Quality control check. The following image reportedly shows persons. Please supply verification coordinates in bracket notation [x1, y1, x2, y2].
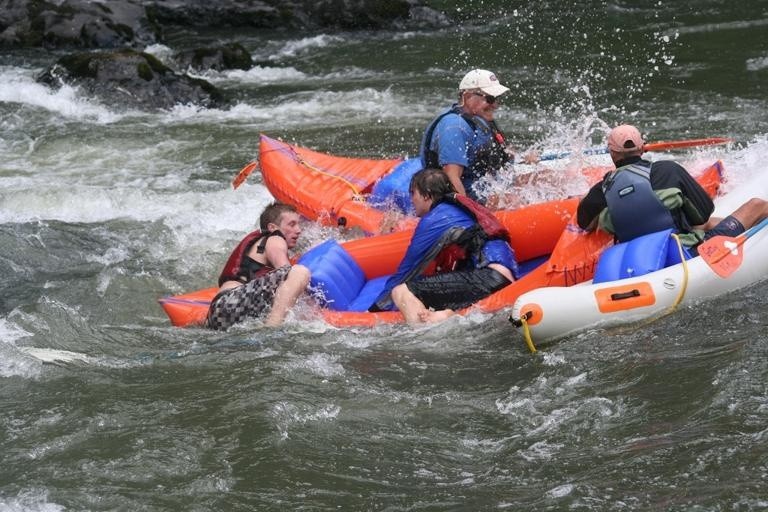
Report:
[419, 68, 542, 206]
[203, 200, 311, 330]
[364, 167, 520, 325]
[575, 123, 768, 256]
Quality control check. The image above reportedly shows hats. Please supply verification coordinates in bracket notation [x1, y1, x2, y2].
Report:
[459, 69, 509, 97]
[608, 124, 644, 151]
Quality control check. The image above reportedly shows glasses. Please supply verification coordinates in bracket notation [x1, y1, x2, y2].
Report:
[473, 92, 495, 104]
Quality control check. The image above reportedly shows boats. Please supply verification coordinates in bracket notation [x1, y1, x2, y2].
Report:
[508, 216, 767, 342]
[155, 192, 615, 332]
[259, 133, 724, 238]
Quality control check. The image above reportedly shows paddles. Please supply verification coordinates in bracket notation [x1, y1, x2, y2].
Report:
[541, 136, 733, 159]
[698, 217, 768, 277]
